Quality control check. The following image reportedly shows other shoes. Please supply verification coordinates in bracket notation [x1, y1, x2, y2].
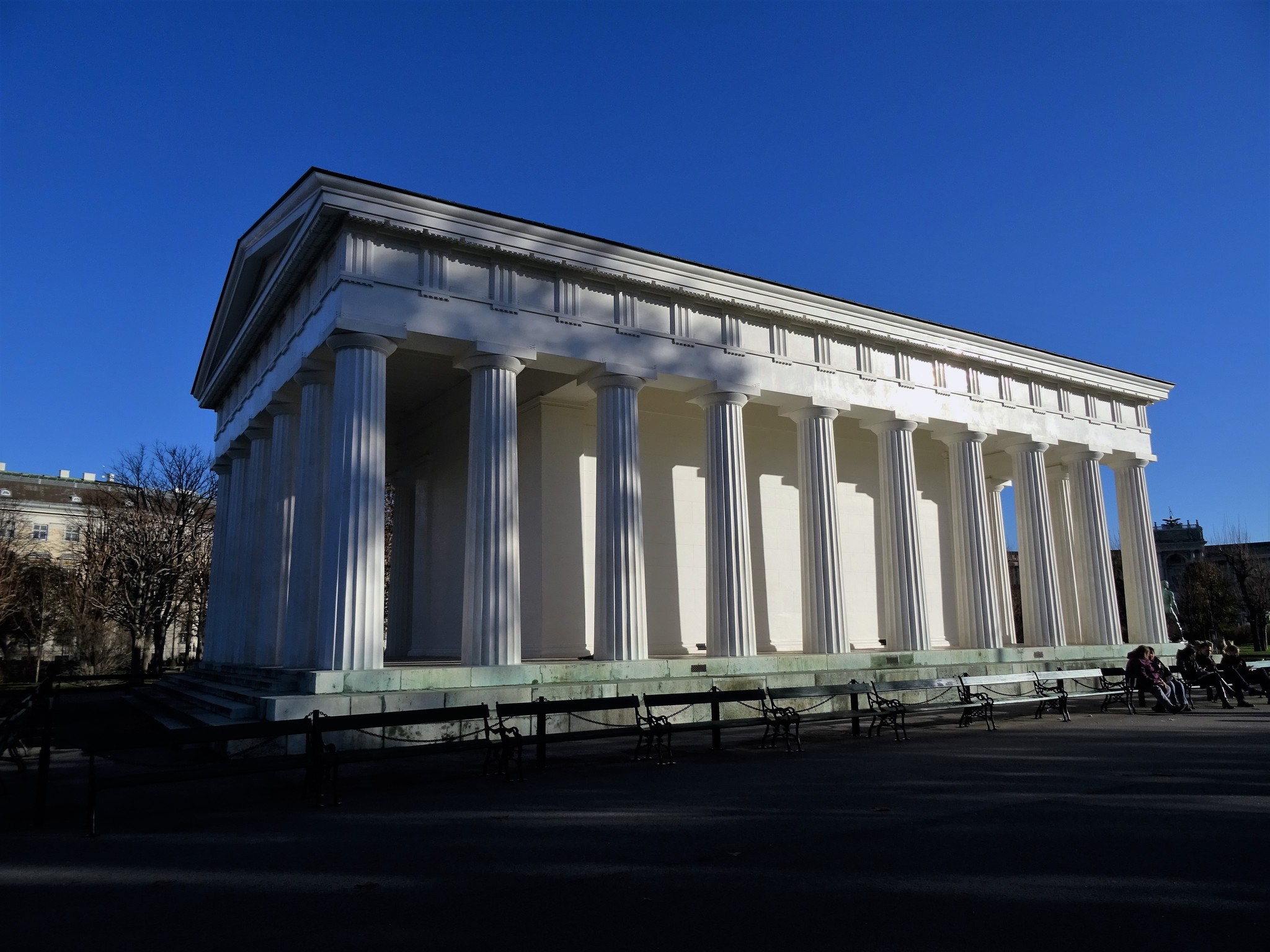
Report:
[1268, 698, 1270, 704]
[1237, 700, 1255, 707]
[1171, 705, 1186, 715]
[1248, 687, 1266, 696]
[1152, 705, 1166, 712]
[1184, 706, 1192, 712]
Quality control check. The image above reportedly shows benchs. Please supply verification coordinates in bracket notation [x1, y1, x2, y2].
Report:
[307, 657, 1269, 798]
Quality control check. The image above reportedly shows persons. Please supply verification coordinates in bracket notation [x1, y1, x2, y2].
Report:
[1177, 640, 1270, 709]
[1124, 645, 1192, 714]
[1161, 580, 1189, 643]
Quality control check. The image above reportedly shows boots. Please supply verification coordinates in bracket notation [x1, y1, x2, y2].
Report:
[1220, 678, 1237, 699]
[1217, 690, 1235, 709]
